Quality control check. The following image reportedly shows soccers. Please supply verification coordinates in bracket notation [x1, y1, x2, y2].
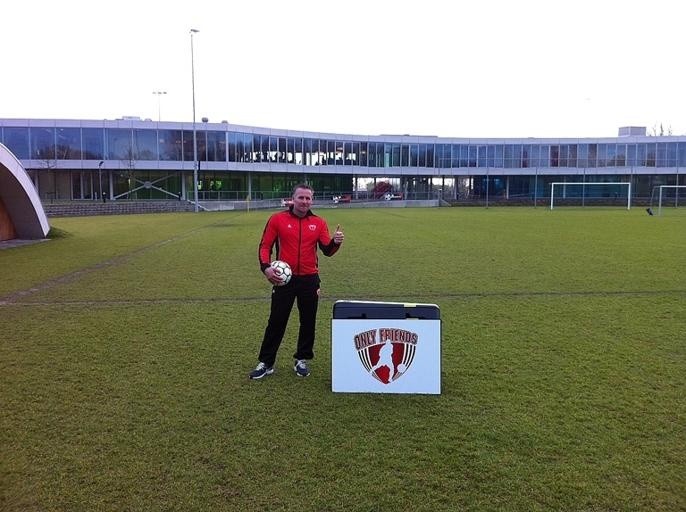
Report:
[270, 260, 292, 286]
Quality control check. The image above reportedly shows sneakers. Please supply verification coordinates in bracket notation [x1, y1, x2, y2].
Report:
[248, 362, 274, 379]
[294, 359, 310, 377]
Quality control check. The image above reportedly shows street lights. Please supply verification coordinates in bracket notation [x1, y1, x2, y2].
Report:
[98, 159, 106, 204]
[189, 28, 201, 213]
[151, 91, 168, 121]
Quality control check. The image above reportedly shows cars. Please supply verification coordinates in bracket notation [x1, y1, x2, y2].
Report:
[280, 198, 293, 207]
[384, 192, 405, 201]
[332, 194, 352, 204]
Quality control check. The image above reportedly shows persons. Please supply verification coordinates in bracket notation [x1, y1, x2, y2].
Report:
[247, 185, 344, 379]
